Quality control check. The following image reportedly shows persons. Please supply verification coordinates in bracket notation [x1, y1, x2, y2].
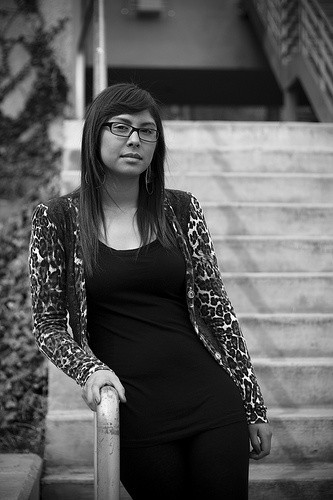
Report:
[29, 83, 271, 500]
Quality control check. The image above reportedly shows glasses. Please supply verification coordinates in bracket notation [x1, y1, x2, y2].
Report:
[103, 122, 159, 142]
